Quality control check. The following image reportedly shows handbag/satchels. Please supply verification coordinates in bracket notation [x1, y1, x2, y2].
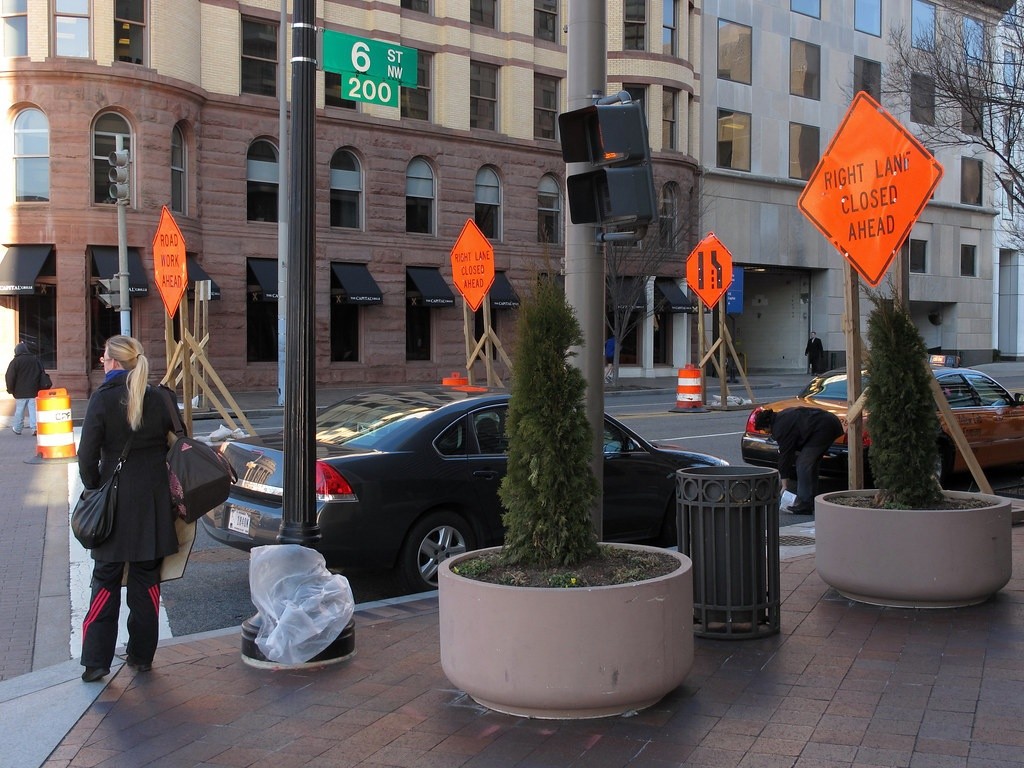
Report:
[37, 358, 52, 390]
[71, 463, 122, 549]
[157, 385, 238, 524]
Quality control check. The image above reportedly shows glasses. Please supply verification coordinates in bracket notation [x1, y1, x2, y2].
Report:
[100, 357, 117, 362]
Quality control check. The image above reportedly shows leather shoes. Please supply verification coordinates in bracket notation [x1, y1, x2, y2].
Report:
[786, 500, 813, 515]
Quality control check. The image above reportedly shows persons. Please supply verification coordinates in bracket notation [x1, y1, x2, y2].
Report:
[755, 407, 846, 514]
[78, 336, 189, 682]
[6, 343, 45, 435]
[805, 332, 824, 377]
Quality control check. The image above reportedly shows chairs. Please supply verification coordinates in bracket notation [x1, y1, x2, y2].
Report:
[476, 418, 504, 454]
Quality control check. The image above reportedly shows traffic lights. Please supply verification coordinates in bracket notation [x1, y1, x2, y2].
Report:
[108, 150, 127, 199]
[95, 278, 120, 308]
[558, 100, 658, 231]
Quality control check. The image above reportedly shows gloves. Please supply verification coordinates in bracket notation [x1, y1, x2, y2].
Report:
[821, 356, 824, 358]
[805, 352, 808, 356]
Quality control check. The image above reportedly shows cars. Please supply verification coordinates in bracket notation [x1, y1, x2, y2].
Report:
[740, 364, 1024, 490]
[199, 381, 733, 590]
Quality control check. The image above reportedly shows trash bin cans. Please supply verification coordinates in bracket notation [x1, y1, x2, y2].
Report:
[675, 467, 782, 641]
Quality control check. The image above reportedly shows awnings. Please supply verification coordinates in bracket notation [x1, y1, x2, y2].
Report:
[607, 276, 693, 312]
[0, 243, 521, 310]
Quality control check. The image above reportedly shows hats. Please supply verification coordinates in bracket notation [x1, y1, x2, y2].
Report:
[755, 409, 773, 430]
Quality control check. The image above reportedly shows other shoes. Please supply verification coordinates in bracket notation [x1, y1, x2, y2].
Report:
[811, 373, 817, 376]
[32, 430, 36, 436]
[126, 656, 152, 671]
[12, 426, 21, 435]
[605, 377, 612, 383]
[82, 666, 110, 682]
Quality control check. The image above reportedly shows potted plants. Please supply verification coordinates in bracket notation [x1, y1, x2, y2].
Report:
[435, 220, 695, 723]
[812, 283, 1013, 612]
